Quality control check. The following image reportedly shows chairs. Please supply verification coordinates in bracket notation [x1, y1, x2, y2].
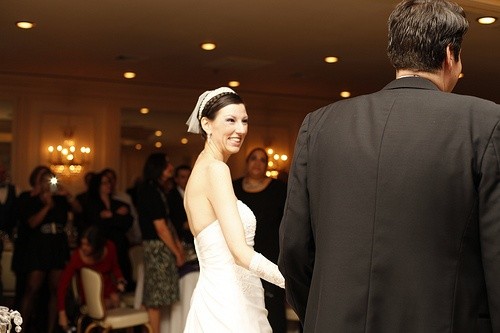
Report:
[77, 267, 154, 333]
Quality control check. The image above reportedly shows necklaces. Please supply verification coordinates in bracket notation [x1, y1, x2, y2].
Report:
[244, 175, 266, 189]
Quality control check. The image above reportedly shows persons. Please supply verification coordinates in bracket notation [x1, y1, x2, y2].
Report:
[231, 147, 287, 327]
[277, 0, 500, 333]
[182, 87, 289, 333]
[0, 144, 199, 333]
[131, 150, 186, 333]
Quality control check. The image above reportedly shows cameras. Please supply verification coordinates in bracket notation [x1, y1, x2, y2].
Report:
[50, 179, 56, 193]
